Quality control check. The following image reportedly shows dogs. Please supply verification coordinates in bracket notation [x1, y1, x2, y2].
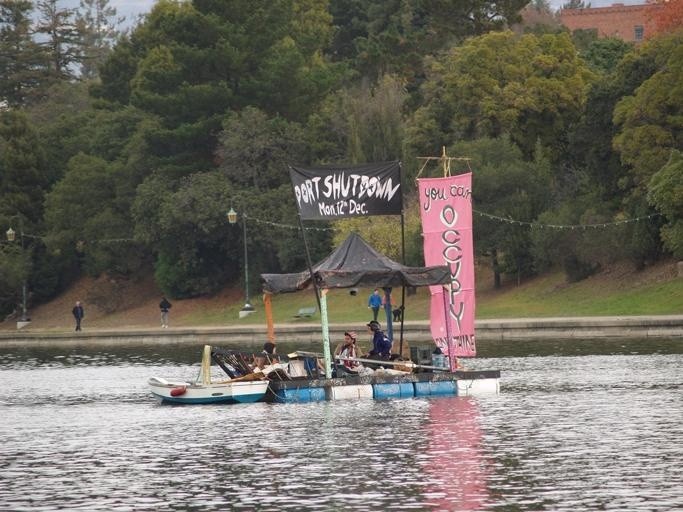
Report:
[392, 305, 405, 322]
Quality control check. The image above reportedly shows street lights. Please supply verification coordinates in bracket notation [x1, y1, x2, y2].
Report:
[227, 207, 256, 311]
[6, 215, 31, 322]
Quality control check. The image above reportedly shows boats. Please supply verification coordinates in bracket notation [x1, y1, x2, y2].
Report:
[148, 146, 501, 405]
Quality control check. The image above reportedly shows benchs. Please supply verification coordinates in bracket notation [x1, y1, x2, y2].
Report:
[293, 308, 317, 320]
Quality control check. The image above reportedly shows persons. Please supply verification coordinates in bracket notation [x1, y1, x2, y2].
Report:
[158, 296, 172, 329]
[331, 330, 362, 378]
[250, 342, 276, 372]
[367, 289, 384, 322]
[359, 320, 392, 372]
[73, 300, 84, 333]
[381, 288, 397, 314]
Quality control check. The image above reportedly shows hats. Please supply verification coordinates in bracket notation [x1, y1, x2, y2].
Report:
[343, 331, 356, 344]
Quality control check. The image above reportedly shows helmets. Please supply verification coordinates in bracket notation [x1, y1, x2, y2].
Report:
[366, 320, 380, 332]
